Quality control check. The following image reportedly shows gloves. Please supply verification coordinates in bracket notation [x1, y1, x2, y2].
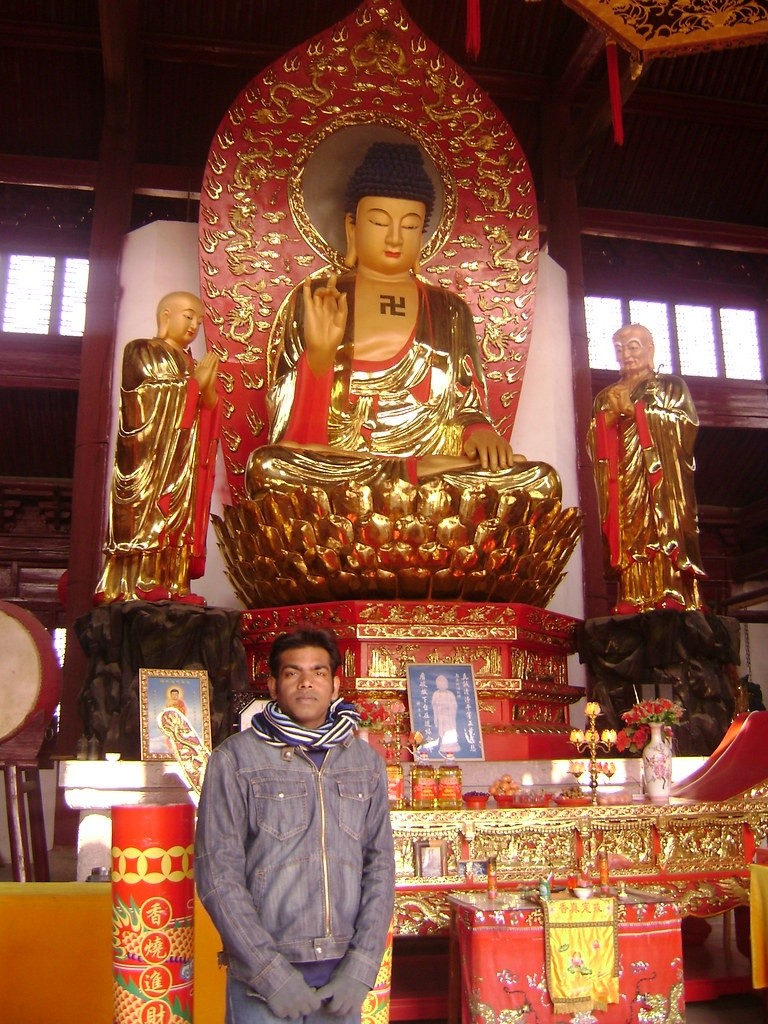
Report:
[267, 971, 321, 1020]
[315, 971, 370, 1017]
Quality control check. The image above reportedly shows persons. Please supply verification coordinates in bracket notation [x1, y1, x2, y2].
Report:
[167, 685, 186, 754]
[428, 674, 463, 754]
[88, 138, 713, 619]
[195, 628, 395, 1024]
